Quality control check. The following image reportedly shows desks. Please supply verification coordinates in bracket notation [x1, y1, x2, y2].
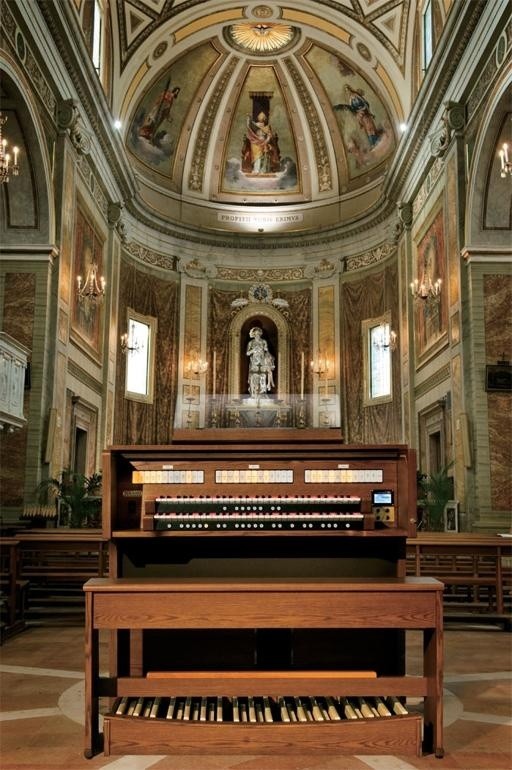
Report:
[81, 576, 445, 760]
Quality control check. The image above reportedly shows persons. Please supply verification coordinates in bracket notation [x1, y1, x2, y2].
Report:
[332, 83, 381, 150]
[245, 109, 277, 173]
[245, 327, 276, 400]
[139, 75, 181, 140]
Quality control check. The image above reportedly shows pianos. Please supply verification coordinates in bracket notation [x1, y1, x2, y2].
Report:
[82, 429, 445, 761]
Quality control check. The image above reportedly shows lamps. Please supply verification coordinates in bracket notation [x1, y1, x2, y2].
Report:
[409, 253, 442, 303]
[75, 252, 106, 304]
